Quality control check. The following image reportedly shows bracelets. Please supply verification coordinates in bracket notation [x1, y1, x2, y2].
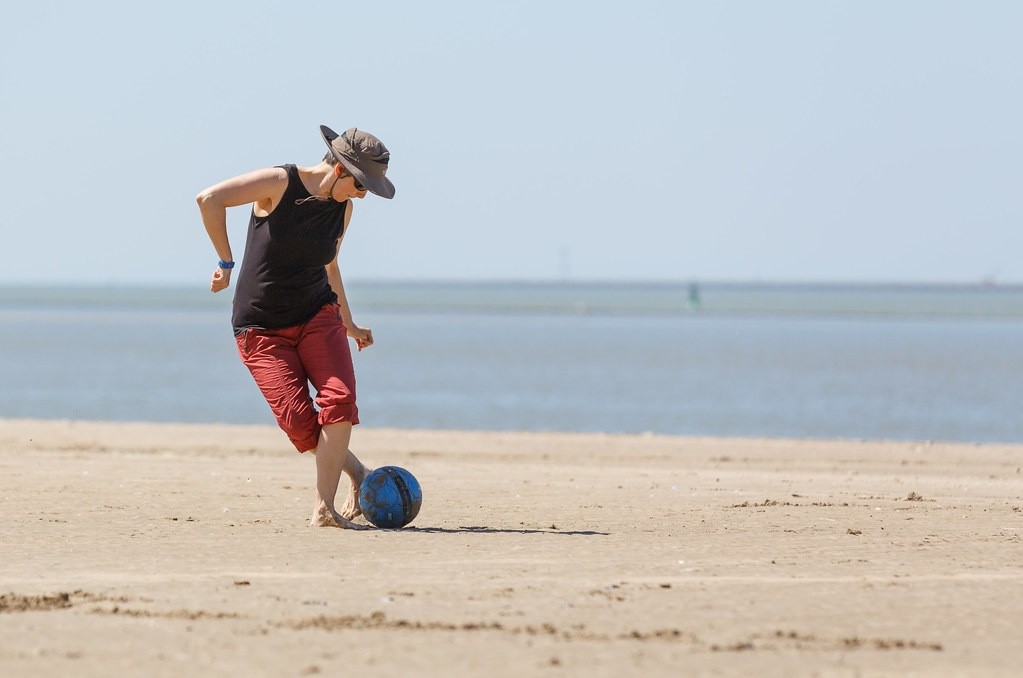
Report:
[218, 260, 235, 268]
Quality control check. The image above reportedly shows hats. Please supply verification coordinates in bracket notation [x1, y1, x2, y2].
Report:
[319, 124, 395, 199]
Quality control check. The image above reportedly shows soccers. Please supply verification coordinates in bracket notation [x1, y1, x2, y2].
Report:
[358, 466, 424, 530]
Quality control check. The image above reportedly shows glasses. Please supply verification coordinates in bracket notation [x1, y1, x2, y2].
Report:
[351, 175, 368, 191]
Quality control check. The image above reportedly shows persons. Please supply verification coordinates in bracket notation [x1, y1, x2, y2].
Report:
[196, 124, 398, 531]
